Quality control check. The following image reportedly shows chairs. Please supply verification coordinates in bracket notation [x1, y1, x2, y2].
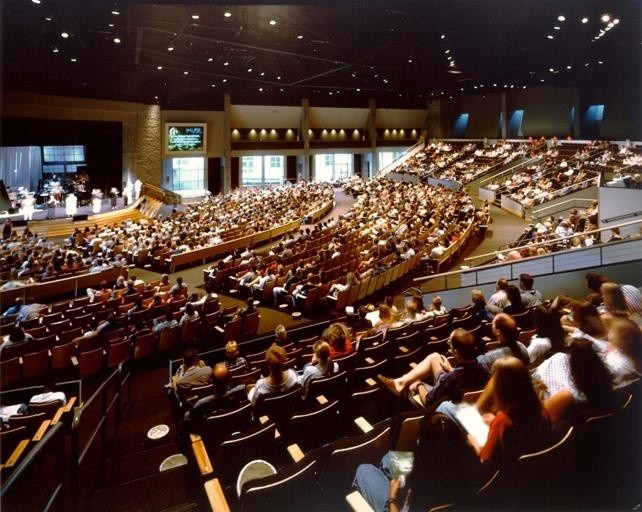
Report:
[229, 367, 263, 386]
[516, 323, 539, 347]
[8, 406, 67, 426]
[26, 393, 77, 414]
[389, 320, 411, 340]
[408, 387, 490, 412]
[481, 334, 493, 344]
[315, 386, 383, 410]
[462, 323, 484, 338]
[346, 482, 466, 512]
[4, 239, 129, 301]
[413, 318, 434, 329]
[297, 335, 319, 347]
[299, 349, 319, 365]
[425, 136, 642, 272]
[353, 411, 425, 446]
[468, 468, 506, 504]
[363, 345, 425, 367]
[5, 434, 30, 471]
[409, 355, 457, 372]
[434, 312, 452, 322]
[187, 422, 281, 477]
[201, 459, 317, 512]
[453, 300, 473, 315]
[187, 378, 234, 397]
[257, 397, 340, 442]
[330, 351, 360, 367]
[268, 356, 299, 376]
[199, 400, 253, 427]
[398, 335, 456, 358]
[5, 417, 52, 444]
[6, 281, 266, 378]
[74, 136, 424, 306]
[352, 356, 388, 380]
[507, 307, 535, 320]
[511, 421, 577, 469]
[284, 425, 393, 464]
[356, 331, 384, 349]
[260, 384, 305, 411]
[582, 387, 634, 436]
[306, 368, 351, 391]
[277, 343, 296, 350]
[363, 374, 385, 386]
[446, 347, 459, 355]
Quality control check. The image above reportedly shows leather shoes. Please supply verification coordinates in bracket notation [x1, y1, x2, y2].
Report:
[377, 374, 402, 396]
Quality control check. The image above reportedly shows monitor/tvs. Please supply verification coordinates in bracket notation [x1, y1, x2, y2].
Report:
[164, 122, 208, 155]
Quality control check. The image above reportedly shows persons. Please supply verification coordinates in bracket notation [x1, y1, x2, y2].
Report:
[352, 292, 447, 335]
[301, 339, 339, 395]
[0, 217, 125, 290]
[519, 273, 544, 309]
[175, 349, 212, 400]
[532, 338, 617, 419]
[29, 378, 67, 406]
[351, 411, 483, 512]
[392, 135, 642, 207]
[486, 278, 508, 319]
[377, 329, 488, 411]
[562, 319, 642, 383]
[91, 188, 103, 213]
[109, 187, 119, 210]
[433, 355, 552, 467]
[0, 273, 219, 361]
[247, 344, 301, 407]
[506, 200, 622, 262]
[476, 312, 530, 367]
[502, 284, 525, 315]
[223, 340, 246, 370]
[192, 362, 245, 414]
[533, 281, 642, 340]
[64, 214, 251, 271]
[337, 173, 490, 266]
[273, 324, 289, 347]
[202, 223, 422, 316]
[170, 178, 334, 235]
[464, 290, 486, 315]
[21, 194, 36, 220]
[324, 324, 353, 359]
[46, 194, 57, 219]
